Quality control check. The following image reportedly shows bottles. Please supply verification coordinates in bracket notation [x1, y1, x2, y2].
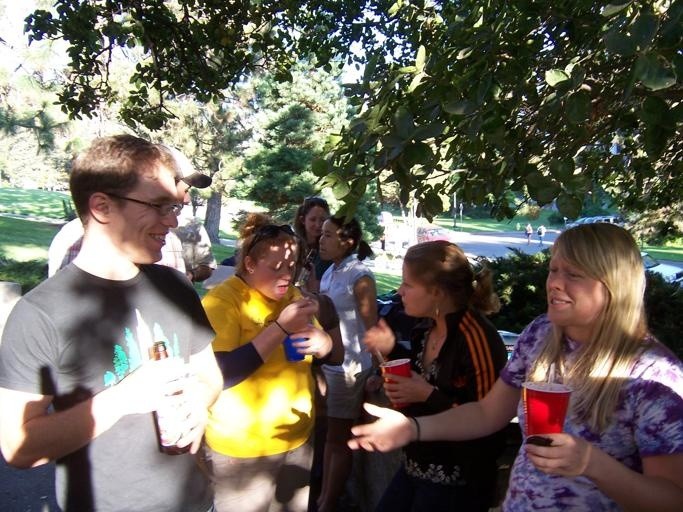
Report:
[153, 341, 192, 455]
[293, 249, 314, 288]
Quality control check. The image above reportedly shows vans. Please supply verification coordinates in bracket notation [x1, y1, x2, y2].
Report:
[416, 225, 452, 245]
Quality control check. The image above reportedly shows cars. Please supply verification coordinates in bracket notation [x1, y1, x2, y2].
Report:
[634, 251, 683, 287]
[561, 215, 627, 232]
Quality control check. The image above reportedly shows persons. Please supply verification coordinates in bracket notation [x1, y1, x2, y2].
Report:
[169, 210, 217, 287]
[45, 141, 212, 280]
[280, 222, 345, 512]
[537, 223, 547, 247]
[345, 221, 682, 511]
[294, 195, 336, 281]
[361, 240, 510, 511]
[308, 212, 382, 512]
[0, 135, 227, 512]
[525, 223, 533, 245]
[201, 212, 334, 512]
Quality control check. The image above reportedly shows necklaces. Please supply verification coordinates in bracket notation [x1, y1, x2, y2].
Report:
[430, 332, 444, 352]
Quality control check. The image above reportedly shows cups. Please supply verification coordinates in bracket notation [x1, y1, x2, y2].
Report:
[521, 382, 572, 434]
[283, 333, 305, 362]
[379, 358, 414, 408]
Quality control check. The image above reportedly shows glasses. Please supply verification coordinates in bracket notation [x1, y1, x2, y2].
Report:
[103, 192, 184, 216]
[246, 223, 294, 256]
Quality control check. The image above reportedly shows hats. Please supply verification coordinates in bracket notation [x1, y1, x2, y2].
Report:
[162, 144, 212, 189]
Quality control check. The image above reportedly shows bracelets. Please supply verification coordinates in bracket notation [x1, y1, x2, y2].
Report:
[273, 319, 290, 339]
[188, 270, 197, 282]
[411, 416, 421, 443]
